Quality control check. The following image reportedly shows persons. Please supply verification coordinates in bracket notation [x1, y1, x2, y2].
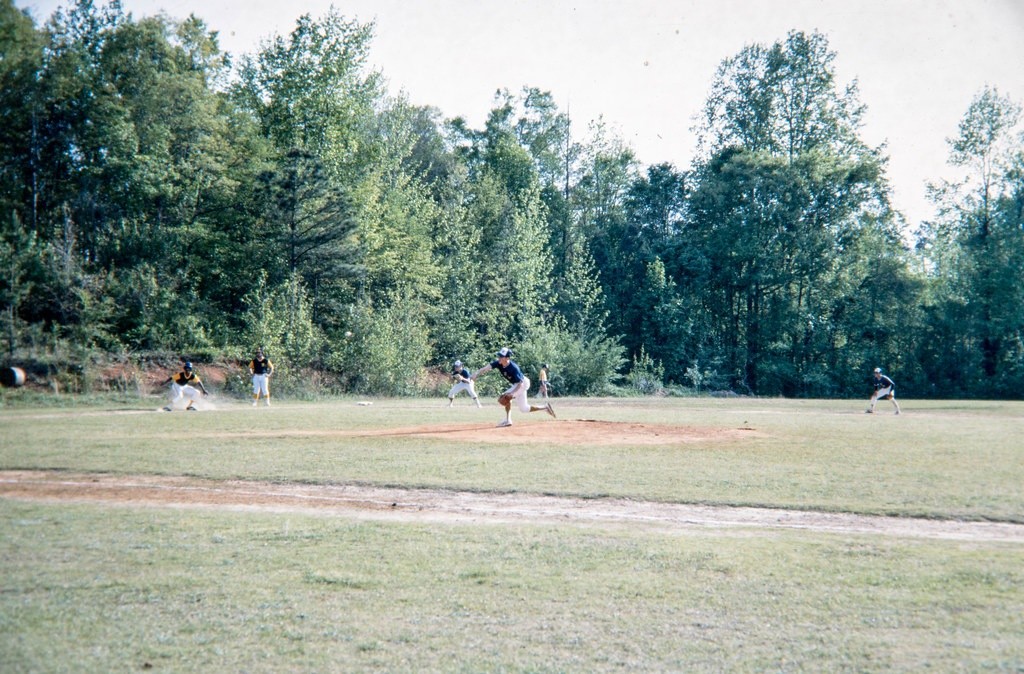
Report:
[865, 368, 900, 415]
[249, 347, 273, 407]
[534, 364, 549, 399]
[160, 362, 208, 411]
[446, 362, 482, 408]
[472, 347, 556, 427]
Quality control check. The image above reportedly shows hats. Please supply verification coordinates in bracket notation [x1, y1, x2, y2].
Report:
[455, 360, 462, 367]
[184, 362, 193, 369]
[255, 348, 263, 352]
[543, 364, 549, 369]
[874, 368, 882, 373]
[497, 348, 513, 357]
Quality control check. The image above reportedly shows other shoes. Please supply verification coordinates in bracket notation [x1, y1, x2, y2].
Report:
[163, 406, 171, 411]
[253, 403, 257, 407]
[546, 403, 556, 419]
[865, 409, 874, 413]
[895, 410, 900, 415]
[496, 421, 512, 427]
[264, 403, 271, 406]
[187, 407, 197, 410]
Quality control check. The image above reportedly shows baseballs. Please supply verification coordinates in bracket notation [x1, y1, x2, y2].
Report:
[345, 331, 351, 337]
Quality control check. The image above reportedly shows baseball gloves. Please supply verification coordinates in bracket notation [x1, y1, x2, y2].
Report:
[454, 373, 463, 381]
[498, 394, 512, 406]
